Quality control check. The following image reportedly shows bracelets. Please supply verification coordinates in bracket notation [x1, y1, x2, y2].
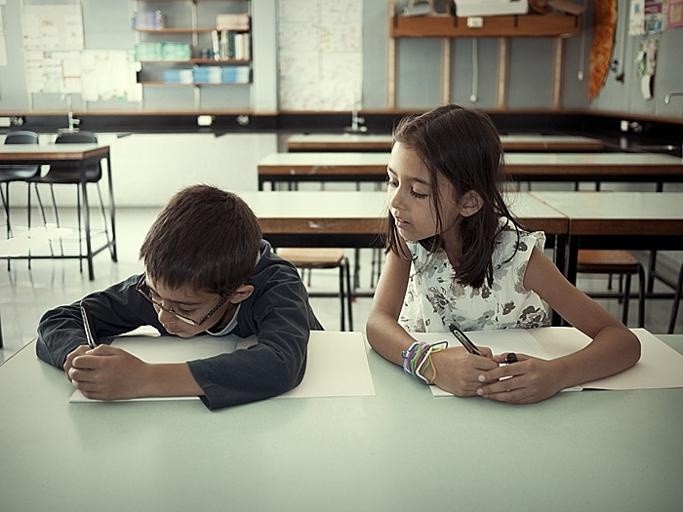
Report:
[400, 339, 448, 385]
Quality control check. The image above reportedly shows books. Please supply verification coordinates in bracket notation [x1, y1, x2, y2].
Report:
[162, 64, 250, 85]
[406, 325, 683, 398]
[67, 330, 377, 402]
[211, 30, 250, 61]
[131, 9, 166, 28]
[215, 12, 249, 32]
[134, 42, 190, 60]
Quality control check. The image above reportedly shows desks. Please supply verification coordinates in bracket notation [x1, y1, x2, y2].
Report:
[1, 328, 683, 510]
[0, 144, 118, 282]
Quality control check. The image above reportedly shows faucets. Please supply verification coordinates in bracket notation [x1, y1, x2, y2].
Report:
[57, 110, 80, 134]
[342, 101, 367, 134]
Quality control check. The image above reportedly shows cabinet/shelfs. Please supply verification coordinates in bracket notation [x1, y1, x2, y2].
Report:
[134, 27, 251, 87]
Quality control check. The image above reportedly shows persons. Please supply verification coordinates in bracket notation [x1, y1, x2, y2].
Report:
[34, 182, 324, 411]
[365, 102, 643, 405]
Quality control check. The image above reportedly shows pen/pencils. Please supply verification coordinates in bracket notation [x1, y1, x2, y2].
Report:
[80, 305, 96, 349]
[448, 323, 481, 356]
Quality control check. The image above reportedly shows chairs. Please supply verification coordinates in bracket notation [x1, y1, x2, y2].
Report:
[26, 131, 115, 272]
[0, 131, 55, 274]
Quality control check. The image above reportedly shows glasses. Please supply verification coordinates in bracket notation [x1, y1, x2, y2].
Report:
[135, 273, 249, 326]
